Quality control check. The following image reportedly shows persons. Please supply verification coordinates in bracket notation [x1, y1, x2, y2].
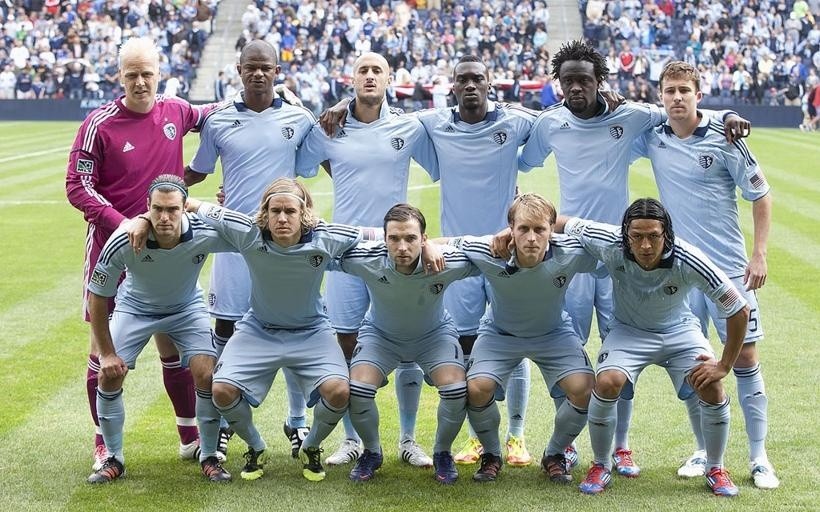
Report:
[542, 75, 561, 108]
[178, 39, 333, 461]
[65, 36, 306, 473]
[428, 193, 602, 486]
[323, 203, 483, 485]
[555, 81, 564, 101]
[125, 175, 446, 484]
[512, 38, 751, 479]
[84, 174, 245, 485]
[213, 1, 550, 114]
[487, 194, 751, 499]
[316, 54, 628, 467]
[1, 1, 220, 100]
[630, 59, 785, 490]
[576, 1, 819, 133]
[216, 51, 520, 469]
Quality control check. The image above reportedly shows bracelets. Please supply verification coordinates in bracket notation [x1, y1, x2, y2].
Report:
[138, 215, 152, 225]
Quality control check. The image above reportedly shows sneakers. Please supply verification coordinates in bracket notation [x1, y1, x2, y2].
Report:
[431, 451, 459, 484]
[749, 461, 780, 488]
[705, 463, 738, 495]
[611, 447, 639, 477]
[283, 422, 311, 460]
[453, 436, 484, 465]
[216, 426, 235, 456]
[505, 432, 531, 466]
[92, 445, 108, 471]
[298, 443, 326, 481]
[473, 453, 503, 482]
[179, 436, 227, 462]
[542, 448, 573, 484]
[325, 439, 364, 465]
[677, 450, 707, 476]
[87, 455, 126, 483]
[563, 444, 578, 471]
[349, 446, 383, 482]
[199, 452, 232, 481]
[240, 446, 271, 480]
[578, 461, 611, 494]
[398, 434, 433, 467]
